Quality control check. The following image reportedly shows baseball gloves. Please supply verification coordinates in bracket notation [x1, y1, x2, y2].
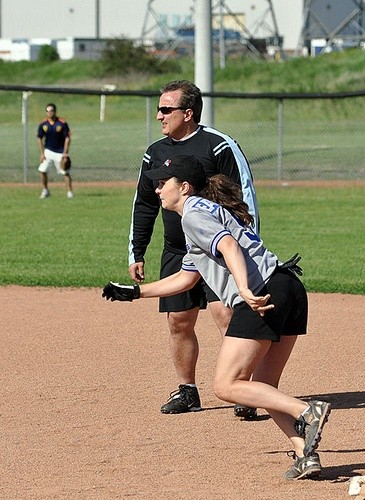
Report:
[58, 155, 71, 171]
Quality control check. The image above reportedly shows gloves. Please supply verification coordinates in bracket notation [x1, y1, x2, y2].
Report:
[281, 253, 303, 276]
[102, 280, 140, 302]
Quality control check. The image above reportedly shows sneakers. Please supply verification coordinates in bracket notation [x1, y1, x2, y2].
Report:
[284, 450, 322, 480]
[39, 188, 49, 200]
[294, 400, 331, 458]
[234, 403, 257, 418]
[67, 191, 73, 199]
[160, 384, 202, 414]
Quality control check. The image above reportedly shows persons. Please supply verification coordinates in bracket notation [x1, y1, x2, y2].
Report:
[102, 155, 332, 480]
[37, 104, 74, 200]
[129, 80, 261, 417]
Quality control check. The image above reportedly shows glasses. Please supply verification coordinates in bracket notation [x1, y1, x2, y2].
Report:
[46, 109, 54, 112]
[157, 105, 186, 114]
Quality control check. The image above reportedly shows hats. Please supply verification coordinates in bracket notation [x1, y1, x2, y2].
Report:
[144, 156, 207, 180]
